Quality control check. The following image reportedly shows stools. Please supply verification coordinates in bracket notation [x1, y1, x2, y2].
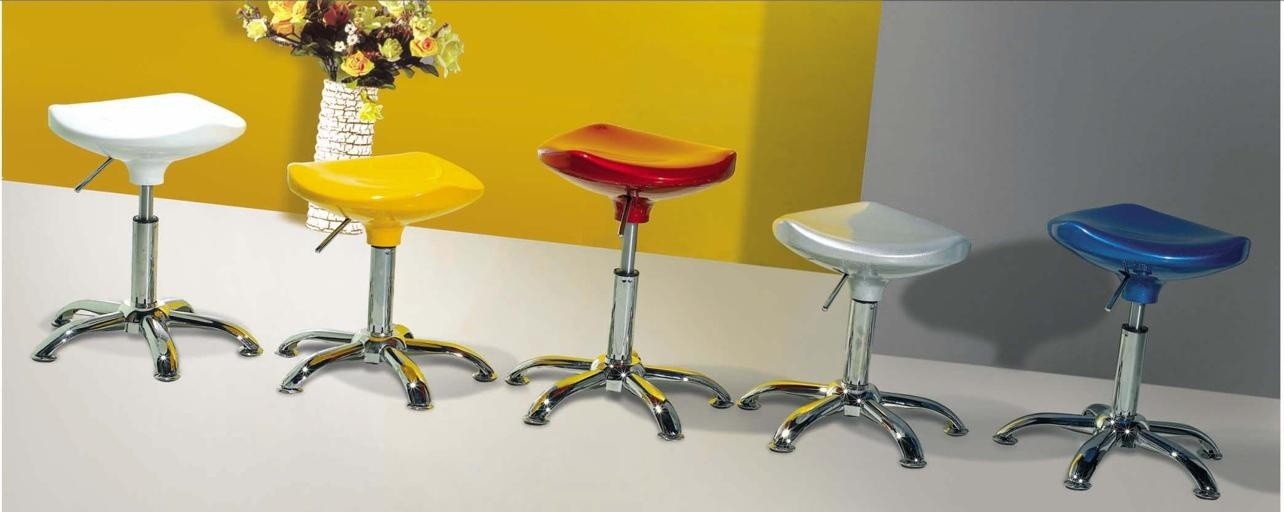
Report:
[737, 200, 972, 469]
[505, 120, 736, 441]
[988, 204, 1250, 498]
[276, 150, 494, 412]
[29, 92, 264, 379]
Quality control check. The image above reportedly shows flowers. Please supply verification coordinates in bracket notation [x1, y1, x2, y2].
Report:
[236, 0, 464, 121]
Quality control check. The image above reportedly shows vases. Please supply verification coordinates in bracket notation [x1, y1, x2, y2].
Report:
[303, 79, 379, 234]
